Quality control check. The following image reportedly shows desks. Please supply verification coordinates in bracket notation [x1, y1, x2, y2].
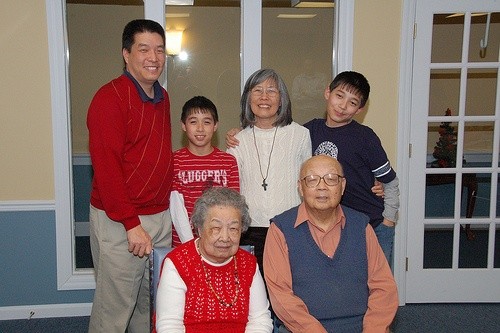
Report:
[426, 163, 477, 240]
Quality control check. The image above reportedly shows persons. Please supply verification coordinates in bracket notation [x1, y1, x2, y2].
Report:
[86, 18, 172, 333]
[150, 186, 274, 333]
[226, 68, 313, 318]
[171, 96, 240, 248]
[223, 70, 400, 267]
[263, 154, 399, 333]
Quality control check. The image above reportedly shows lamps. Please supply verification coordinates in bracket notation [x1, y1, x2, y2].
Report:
[292, 0, 335, 9]
[165, 29, 183, 57]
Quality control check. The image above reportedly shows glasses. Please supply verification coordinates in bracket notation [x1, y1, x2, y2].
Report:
[301, 173, 343, 188]
[251, 87, 281, 97]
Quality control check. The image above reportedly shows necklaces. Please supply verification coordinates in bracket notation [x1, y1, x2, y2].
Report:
[253, 125, 279, 191]
[197, 241, 239, 307]
